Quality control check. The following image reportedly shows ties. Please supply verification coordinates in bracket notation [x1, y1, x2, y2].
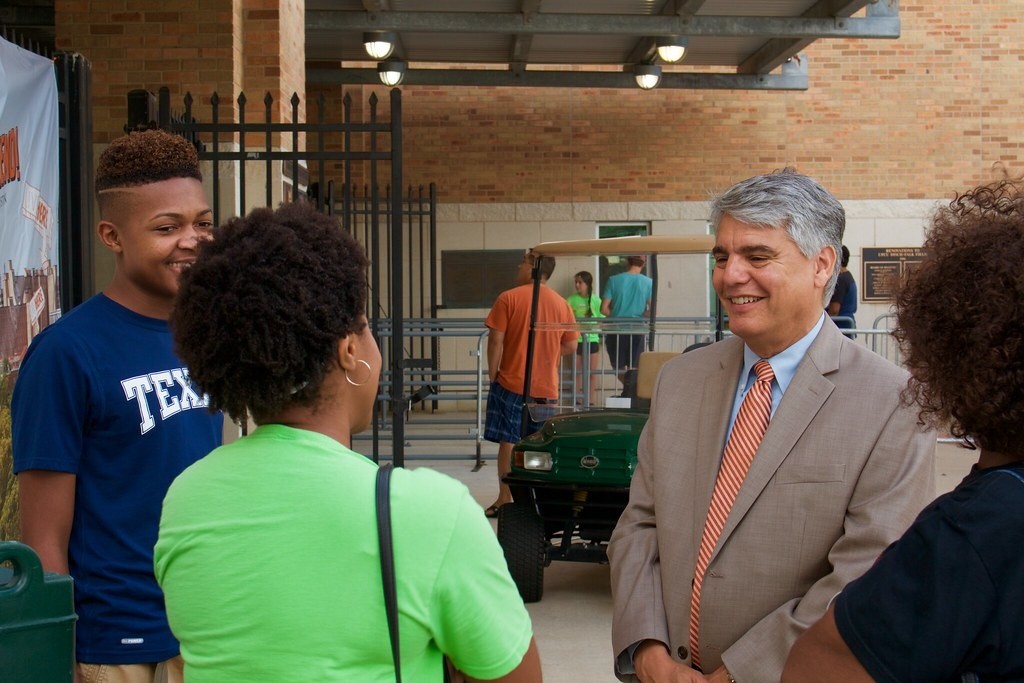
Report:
[688, 359, 775, 669]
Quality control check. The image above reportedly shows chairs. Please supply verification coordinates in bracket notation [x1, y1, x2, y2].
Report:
[621, 370, 651, 412]
[637, 352, 684, 398]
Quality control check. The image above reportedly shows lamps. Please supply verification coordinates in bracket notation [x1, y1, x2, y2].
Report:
[376, 60, 409, 87]
[657, 36, 687, 63]
[361, 28, 395, 60]
[633, 65, 662, 90]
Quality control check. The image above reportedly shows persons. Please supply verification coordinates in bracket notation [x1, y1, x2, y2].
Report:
[599, 253, 653, 395]
[565, 270, 607, 411]
[822, 244, 859, 342]
[152, 195, 544, 682]
[483, 246, 582, 518]
[606, 164, 938, 683]
[775, 174, 1024, 683]
[7, 127, 225, 682]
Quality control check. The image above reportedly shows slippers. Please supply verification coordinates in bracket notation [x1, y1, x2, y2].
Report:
[484, 506, 500, 519]
